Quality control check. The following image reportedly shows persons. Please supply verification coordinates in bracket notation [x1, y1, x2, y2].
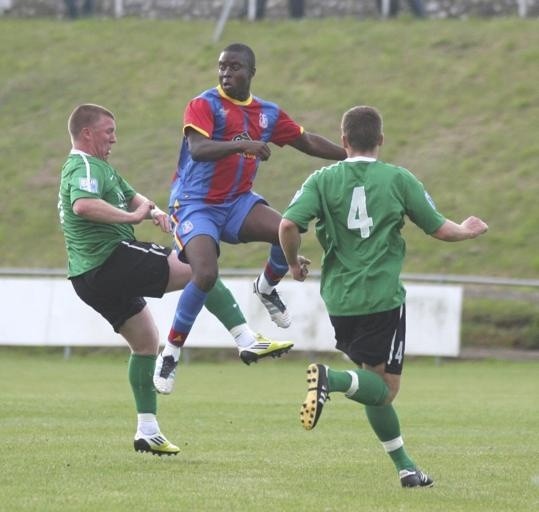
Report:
[278, 106, 489, 488]
[152, 44, 348, 395]
[58, 103, 294, 454]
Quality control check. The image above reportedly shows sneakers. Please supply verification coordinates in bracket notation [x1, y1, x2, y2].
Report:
[153, 352, 180, 394]
[253, 275, 291, 328]
[399, 469, 433, 488]
[238, 334, 294, 365]
[299, 364, 330, 430]
[134, 428, 180, 455]
